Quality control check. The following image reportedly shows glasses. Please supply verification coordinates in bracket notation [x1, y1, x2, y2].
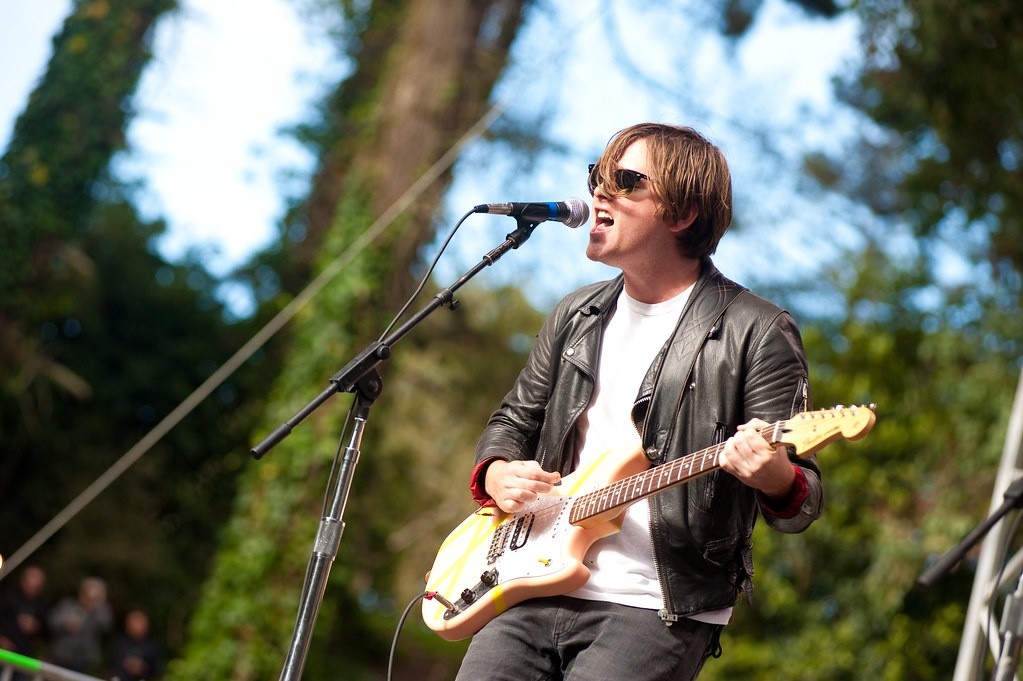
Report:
[587, 163, 659, 195]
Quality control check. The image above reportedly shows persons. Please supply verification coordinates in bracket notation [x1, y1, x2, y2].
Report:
[454, 122, 827, 681]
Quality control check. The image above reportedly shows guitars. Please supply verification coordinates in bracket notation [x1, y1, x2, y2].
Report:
[422, 401, 877, 642]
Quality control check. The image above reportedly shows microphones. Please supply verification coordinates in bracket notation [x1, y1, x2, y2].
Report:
[474, 197, 590, 229]
